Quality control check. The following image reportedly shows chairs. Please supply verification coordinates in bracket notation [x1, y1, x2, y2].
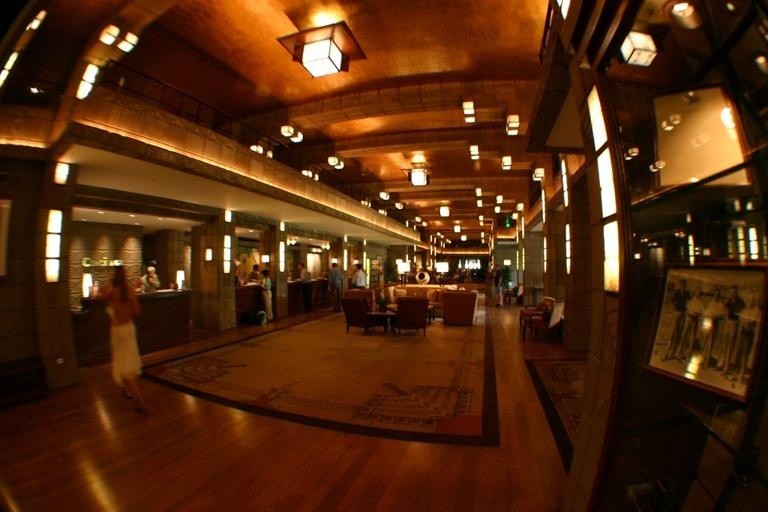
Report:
[343, 283, 478, 335]
[503, 287, 565, 345]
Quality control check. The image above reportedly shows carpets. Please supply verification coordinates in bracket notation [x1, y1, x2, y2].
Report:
[139, 289, 501, 446]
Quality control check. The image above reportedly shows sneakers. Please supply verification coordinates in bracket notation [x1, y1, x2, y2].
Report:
[132, 407, 151, 416]
[122, 390, 133, 401]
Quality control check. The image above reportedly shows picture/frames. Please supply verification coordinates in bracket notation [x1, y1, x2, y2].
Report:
[636, 252, 766, 403]
[642, 82, 761, 198]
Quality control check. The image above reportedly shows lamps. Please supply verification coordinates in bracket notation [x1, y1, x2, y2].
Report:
[619, 29, 658, 66]
[430, 187, 524, 245]
[501, 155, 511, 169]
[621, 87, 714, 194]
[463, 102, 476, 123]
[532, 168, 545, 181]
[470, 145, 480, 160]
[250, 125, 344, 181]
[506, 115, 519, 136]
[276, 20, 368, 78]
[403, 168, 432, 186]
[361, 192, 429, 231]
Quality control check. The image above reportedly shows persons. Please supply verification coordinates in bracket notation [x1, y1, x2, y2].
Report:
[664, 279, 759, 384]
[494, 265, 504, 307]
[327, 263, 343, 312]
[486, 269, 495, 298]
[246, 265, 260, 282]
[97, 266, 149, 413]
[456, 266, 478, 281]
[257, 269, 274, 323]
[140, 266, 161, 293]
[351, 263, 366, 289]
[297, 262, 312, 313]
